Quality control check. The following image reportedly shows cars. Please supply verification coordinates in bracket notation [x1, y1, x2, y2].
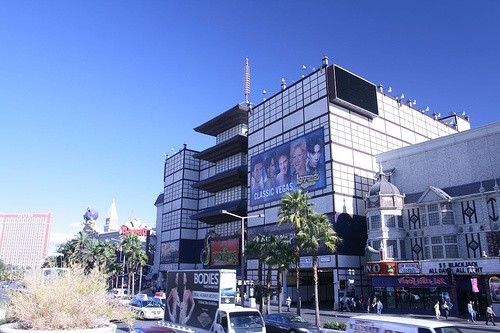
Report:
[127, 292, 166, 321]
[110, 289, 128, 296]
[263, 312, 320, 333]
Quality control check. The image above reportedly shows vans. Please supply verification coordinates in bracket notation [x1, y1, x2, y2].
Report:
[344, 315, 463, 333]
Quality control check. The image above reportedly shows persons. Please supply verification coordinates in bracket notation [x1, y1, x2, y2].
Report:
[286, 296, 291, 311]
[251, 137, 323, 198]
[486, 305, 496, 326]
[434, 301, 441, 320]
[441, 300, 449, 320]
[167, 272, 195, 325]
[339, 296, 383, 314]
[467, 301, 477, 323]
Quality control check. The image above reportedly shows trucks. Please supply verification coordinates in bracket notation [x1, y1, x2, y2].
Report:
[159, 269, 267, 333]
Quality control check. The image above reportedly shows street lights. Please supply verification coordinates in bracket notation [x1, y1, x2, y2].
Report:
[348, 269, 355, 311]
[468, 265, 481, 320]
[219, 209, 265, 306]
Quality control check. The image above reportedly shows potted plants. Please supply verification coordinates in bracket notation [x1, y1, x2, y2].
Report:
[320, 320, 346, 333]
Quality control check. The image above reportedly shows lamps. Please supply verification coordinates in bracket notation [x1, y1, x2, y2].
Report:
[453, 123, 457, 130]
[322, 56, 329, 66]
[377, 84, 441, 120]
[263, 65, 307, 101]
[461, 111, 470, 121]
